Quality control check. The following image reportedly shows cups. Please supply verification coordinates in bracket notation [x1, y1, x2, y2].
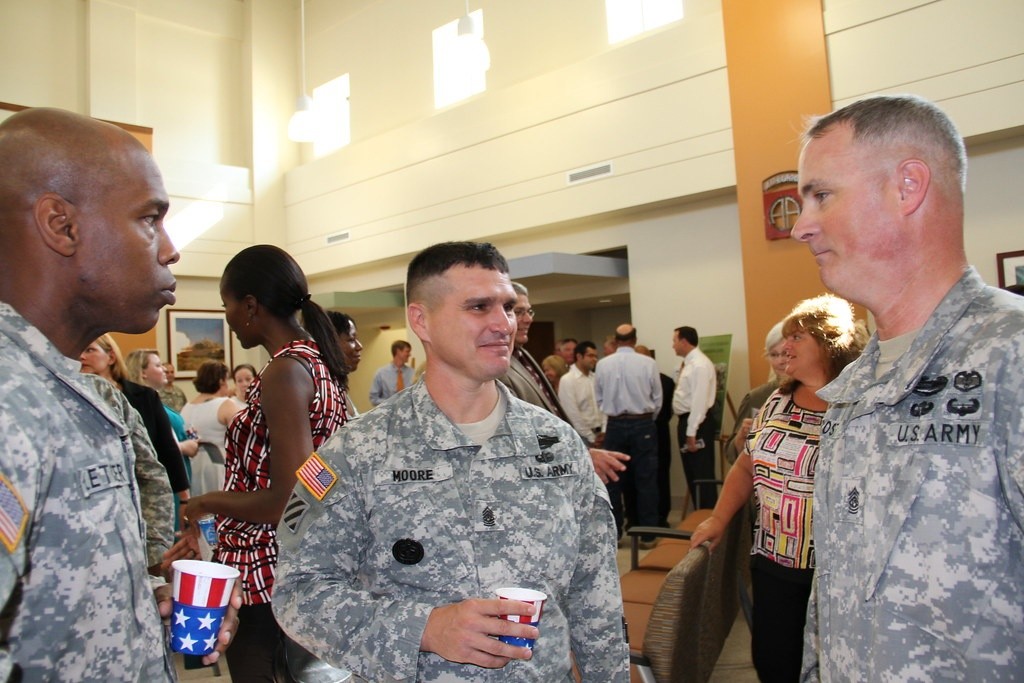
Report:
[196, 513, 218, 549]
[170, 559, 241, 656]
[493, 588, 548, 651]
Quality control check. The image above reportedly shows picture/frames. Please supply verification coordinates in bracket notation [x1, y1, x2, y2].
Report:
[166, 308, 233, 379]
[996, 250, 1024, 288]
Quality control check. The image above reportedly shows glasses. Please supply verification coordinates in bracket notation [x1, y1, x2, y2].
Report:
[513, 309, 535, 317]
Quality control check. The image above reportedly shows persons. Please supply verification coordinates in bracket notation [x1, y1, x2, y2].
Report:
[688, 294, 873, 683]
[271, 241, 630, 683]
[791, 93, 1024, 683]
[160, 244, 348, 683]
[499, 281, 631, 484]
[672, 326, 719, 510]
[78, 333, 193, 537]
[81, 372, 176, 582]
[369, 340, 416, 406]
[0, 107, 243, 683]
[325, 310, 363, 421]
[126, 350, 258, 562]
[542, 323, 675, 549]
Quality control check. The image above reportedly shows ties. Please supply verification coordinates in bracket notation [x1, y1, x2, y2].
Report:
[397, 369, 404, 393]
[515, 349, 564, 419]
[675, 362, 685, 381]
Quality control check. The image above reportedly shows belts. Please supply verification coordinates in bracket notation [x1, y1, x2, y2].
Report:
[608, 413, 653, 421]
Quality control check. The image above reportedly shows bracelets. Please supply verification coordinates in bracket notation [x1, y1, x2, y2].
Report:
[180, 500, 189, 504]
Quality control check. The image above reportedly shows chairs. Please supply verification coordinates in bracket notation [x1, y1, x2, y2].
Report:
[570, 479, 753, 683]
